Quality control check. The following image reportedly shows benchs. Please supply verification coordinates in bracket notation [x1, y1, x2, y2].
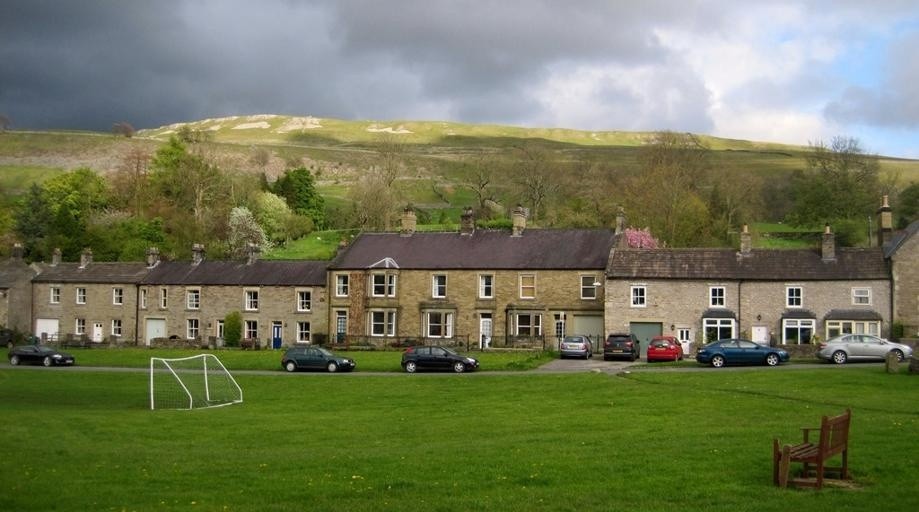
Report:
[769, 404, 854, 489]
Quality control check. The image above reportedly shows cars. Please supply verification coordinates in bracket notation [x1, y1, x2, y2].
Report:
[813, 333, 912, 364]
[401, 345, 479, 373]
[697, 338, 791, 368]
[8, 344, 74, 366]
[647, 335, 683, 361]
[560, 335, 593, 359]
[280, 344, 356, 372]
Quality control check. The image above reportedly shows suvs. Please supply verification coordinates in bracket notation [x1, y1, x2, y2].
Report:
[604, 333, 639, 360]
